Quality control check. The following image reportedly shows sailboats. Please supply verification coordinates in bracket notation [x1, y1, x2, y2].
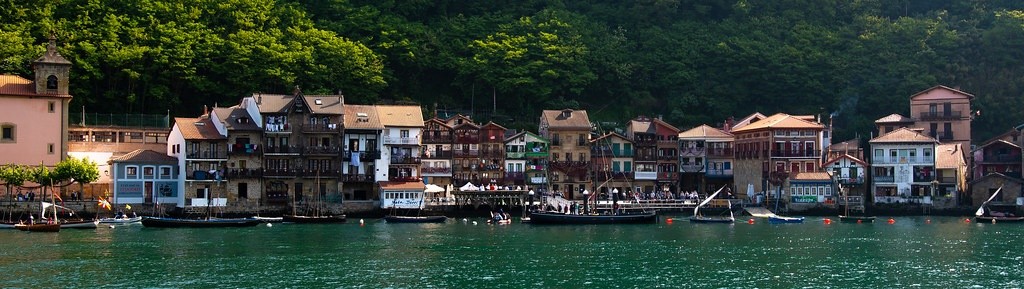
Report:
[527, 119, 661, 225]
[282, 163, 347, 224]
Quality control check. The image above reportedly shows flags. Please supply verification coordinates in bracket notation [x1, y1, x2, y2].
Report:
[98, 196, 111, 211]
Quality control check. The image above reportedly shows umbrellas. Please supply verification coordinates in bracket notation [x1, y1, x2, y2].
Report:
[424, 183, 445, 202]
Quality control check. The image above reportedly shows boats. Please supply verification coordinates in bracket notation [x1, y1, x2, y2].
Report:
[768, 214, 804, 225]
[487, 212, 512, 226]
[140, 178, 262, 228]
[838, 187, 877, 222]
[689, 184, 735, 223]
[0, 160, 143, 229]
[251, 216, 283, 223]
[974, 185, 1024, 224]
[13, 178, 62, 232]
[383, 186, 448, 224]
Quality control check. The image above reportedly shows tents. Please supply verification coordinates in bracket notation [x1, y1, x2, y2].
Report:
[459, 182, 479, 191]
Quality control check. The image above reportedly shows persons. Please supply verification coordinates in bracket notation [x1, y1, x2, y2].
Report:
[453, 182, 528, 205]
[104, 208, 139, 219]
[23, 204, 75, 225]
[494, 208, 511, 220]
[622, 188, 700, 207]
[543, 200, 591, 216]
[550, 189, 566, 198]
[70, 190, 82, 202]
[612, 199, 620, 216]
[15, 188, 35, 202]
